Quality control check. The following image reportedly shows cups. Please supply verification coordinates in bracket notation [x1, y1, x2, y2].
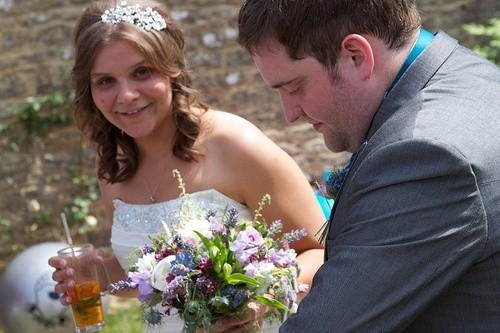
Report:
[58, 243, 106, 333]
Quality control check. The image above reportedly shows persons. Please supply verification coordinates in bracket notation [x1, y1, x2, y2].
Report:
[48, 0, 329, 333]
[235, 0, 499, 332]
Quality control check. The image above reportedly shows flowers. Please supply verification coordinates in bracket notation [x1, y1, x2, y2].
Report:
[313, 163, 353, 246]
[100, 0, 170, 31]
[98, 167, 313, 332]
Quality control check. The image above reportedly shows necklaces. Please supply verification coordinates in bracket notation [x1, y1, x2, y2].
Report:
[134, 143, 171, 204]
